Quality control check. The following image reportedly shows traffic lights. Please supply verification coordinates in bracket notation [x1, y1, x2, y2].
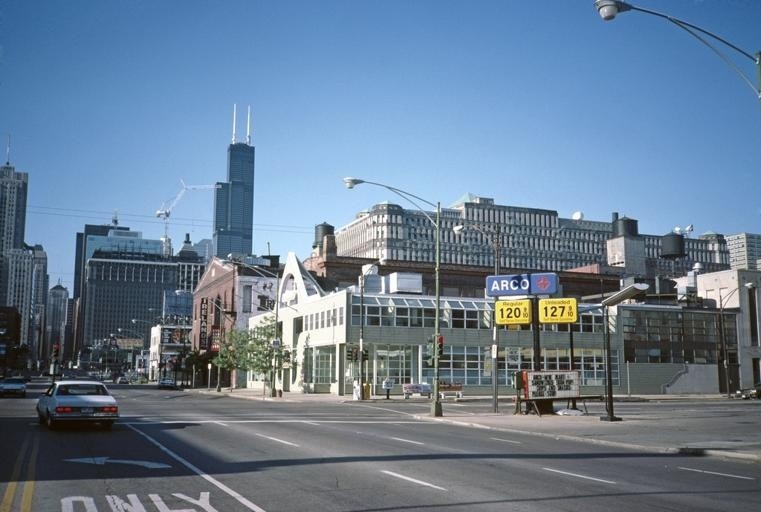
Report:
[346, 351, 352, 360]
[51, 344, 58, 357]
[434, 333, 444, 358]
[425, 334, 434, 358]
[351, 348, 358, 360]
[361, 349, 368, 361]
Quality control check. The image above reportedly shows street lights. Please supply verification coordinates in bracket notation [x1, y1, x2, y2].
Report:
[342, 174, 443, 419]
[91, 306, 179, 386]
[216, 257, 281, 397]
[594, 280, 648, 424]
[452, 220, 502, 414]
[354, 255, 387, 401]
[171, 289, 224, 392]
[595, 0, 761, 98]
[719, 281, 755, 398]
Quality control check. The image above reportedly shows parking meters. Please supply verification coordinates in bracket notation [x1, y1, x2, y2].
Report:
[509, 370, 523, 416]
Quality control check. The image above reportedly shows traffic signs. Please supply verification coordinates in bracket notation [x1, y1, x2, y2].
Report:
[537, 298, 577, 323]
[494, 298, 533, 325]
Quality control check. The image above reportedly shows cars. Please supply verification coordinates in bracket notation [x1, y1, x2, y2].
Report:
[0, 378, 27, 399]
[733, 382, 761, 401]
[33, 378, 119, 433]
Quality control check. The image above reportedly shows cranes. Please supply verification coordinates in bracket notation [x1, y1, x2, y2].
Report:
[153, 183, 223, 240]
